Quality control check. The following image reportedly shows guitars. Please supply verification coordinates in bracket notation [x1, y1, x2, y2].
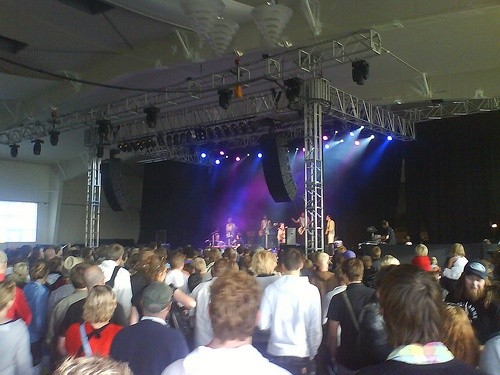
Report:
[258, 224, 277, 237]
[298, 222, 311, 235]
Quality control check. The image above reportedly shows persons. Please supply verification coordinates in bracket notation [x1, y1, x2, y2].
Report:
[291, 212, 310, 244]
[233, 233, 245, 247]
[325, 214, 335, 257]
[210, 229, 225, 246]
[258, 215, 287, 247]
[0, 241, 500, 375]
[373, 219, 395, 247]
[225, 217, 241, 251]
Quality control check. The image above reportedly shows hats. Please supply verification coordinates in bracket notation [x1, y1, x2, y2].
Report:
[142, 284, 171, 313]
[465, 260, 487, 281]
[62, 256, 85, 276]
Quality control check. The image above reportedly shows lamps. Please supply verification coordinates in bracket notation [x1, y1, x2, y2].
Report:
[96, 120, 111, 137]
[218, 87, 233, 108]
[9, 145, 19, 157]
[282, 77, 304, 102]
[351, 60, 369, 85]
[117, 121, 260, 152]
[144, 107, 160, 128]
[32, 139, 44, 155]
[97, 145, 104, 157]
[48, 131, 60, 146]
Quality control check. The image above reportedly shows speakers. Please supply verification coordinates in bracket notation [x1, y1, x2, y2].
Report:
[287, 228, 296, 246]
[101, 160, 133, 211]
[156, 230, 166, 244]
[260, 133, 298, 201]
